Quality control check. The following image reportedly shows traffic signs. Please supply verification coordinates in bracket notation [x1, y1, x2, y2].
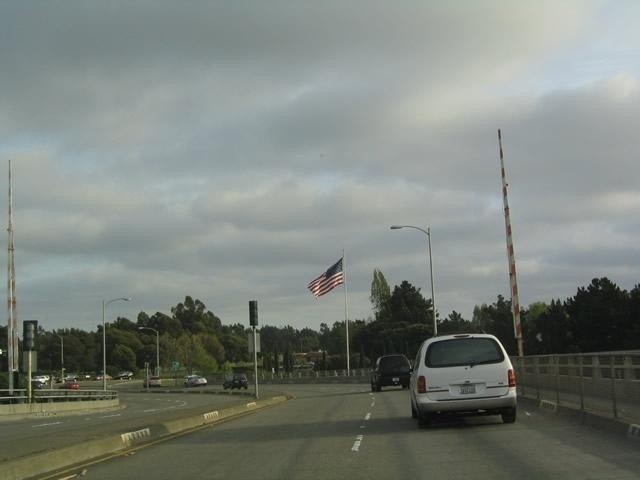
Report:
[172, 362, 182, 367]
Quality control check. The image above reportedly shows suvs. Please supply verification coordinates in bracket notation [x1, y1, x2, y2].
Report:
[368, 353, 413, 393]
[222, 373, 249, 389]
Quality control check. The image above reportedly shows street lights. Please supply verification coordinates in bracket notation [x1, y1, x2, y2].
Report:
[44, 329, 66, 384]
[101, 297, 133, 390]
[389, 222, 439, 339]
[139, 326, 161, 380]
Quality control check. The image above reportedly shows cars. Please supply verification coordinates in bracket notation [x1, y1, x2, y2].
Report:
[142, 375, 162, 388]
[26, 370, 134, 392]
[183, 374, 209, 387]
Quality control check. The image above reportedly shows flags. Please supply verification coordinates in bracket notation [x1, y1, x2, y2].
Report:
[306, 257, 344, 300]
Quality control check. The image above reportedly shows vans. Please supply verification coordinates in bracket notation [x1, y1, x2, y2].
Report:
[409, 330, 517, 429]
